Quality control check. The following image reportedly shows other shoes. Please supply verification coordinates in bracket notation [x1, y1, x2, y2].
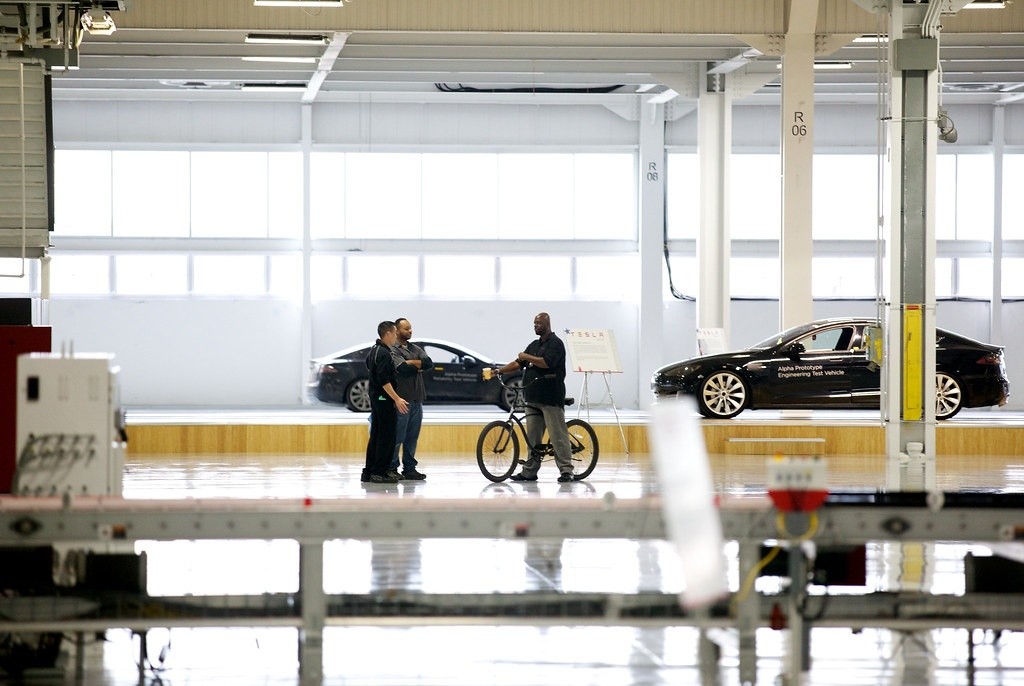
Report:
[370, 471, 398, 483]
[402, 470, 426, 480]
[558, 472, 574, 482]
[387, 468, 406, 480]
[361, 468, 370, 482]
[510, 473, 538, 481]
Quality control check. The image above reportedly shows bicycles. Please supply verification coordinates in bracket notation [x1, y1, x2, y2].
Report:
[475, 367, 600, 482]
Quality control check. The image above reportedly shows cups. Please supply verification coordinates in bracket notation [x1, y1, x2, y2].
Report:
[481, 368, 492, 380]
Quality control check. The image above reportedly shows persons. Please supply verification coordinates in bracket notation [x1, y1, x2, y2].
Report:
[385, 317, 434, 480]
[482, 313, 575, 482]
[360, 322, 410, 483]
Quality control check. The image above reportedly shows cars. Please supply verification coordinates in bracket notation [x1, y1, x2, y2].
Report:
[648, 317, 1010, 421]
[305, 340, 504, 414]
